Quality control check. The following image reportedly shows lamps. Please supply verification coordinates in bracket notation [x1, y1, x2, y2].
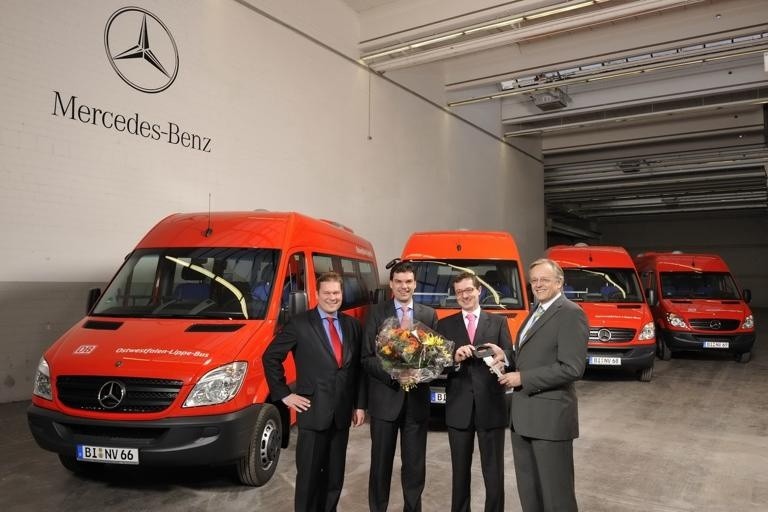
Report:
[448, 44, 768, 113]
[359, 0, 705, 75]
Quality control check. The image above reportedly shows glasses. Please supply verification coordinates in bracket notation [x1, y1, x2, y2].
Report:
[455, 287, 474, 296]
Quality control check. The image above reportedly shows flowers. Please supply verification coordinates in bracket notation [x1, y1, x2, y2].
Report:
[373, 318, 455, 392]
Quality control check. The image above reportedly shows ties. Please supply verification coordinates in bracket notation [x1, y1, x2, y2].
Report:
[327, 316, 342, 368]
[465, 314, 476, 344]
[400, 307, 410, 331]
[521, 307, 543, 343]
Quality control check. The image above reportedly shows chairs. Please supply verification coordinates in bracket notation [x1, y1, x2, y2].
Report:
[563, 285, 575, 299]
[486, 270, 503, 285]
[252, 262, 295, 307]
[600, 285, 616, 296]
[173, 264, 210, 299]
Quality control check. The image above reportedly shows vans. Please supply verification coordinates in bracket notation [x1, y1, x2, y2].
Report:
[635, 249, 757, 364]
[28, 194, 381, 488]
[545, 241, 658, 383]
[386, 228, 535, 405]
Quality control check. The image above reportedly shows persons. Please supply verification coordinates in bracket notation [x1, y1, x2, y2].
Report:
[261, 272, 369, 512]
[360, 261, 438, 511]
[437, 271, 515, 512]
[483, 258, 591, 512]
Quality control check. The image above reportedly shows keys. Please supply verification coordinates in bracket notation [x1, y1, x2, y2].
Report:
[473, 342, 503, 380]
